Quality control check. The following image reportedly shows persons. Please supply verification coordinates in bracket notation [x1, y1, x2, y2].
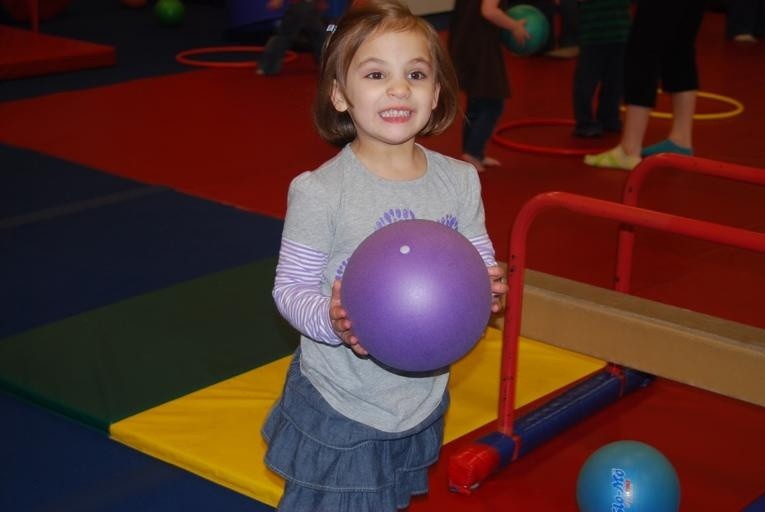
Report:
[448, 0, 533, 173]
[261, 0, 509, 512]
[571, 0, 632, 137]
[584, 0, 704, 170]
